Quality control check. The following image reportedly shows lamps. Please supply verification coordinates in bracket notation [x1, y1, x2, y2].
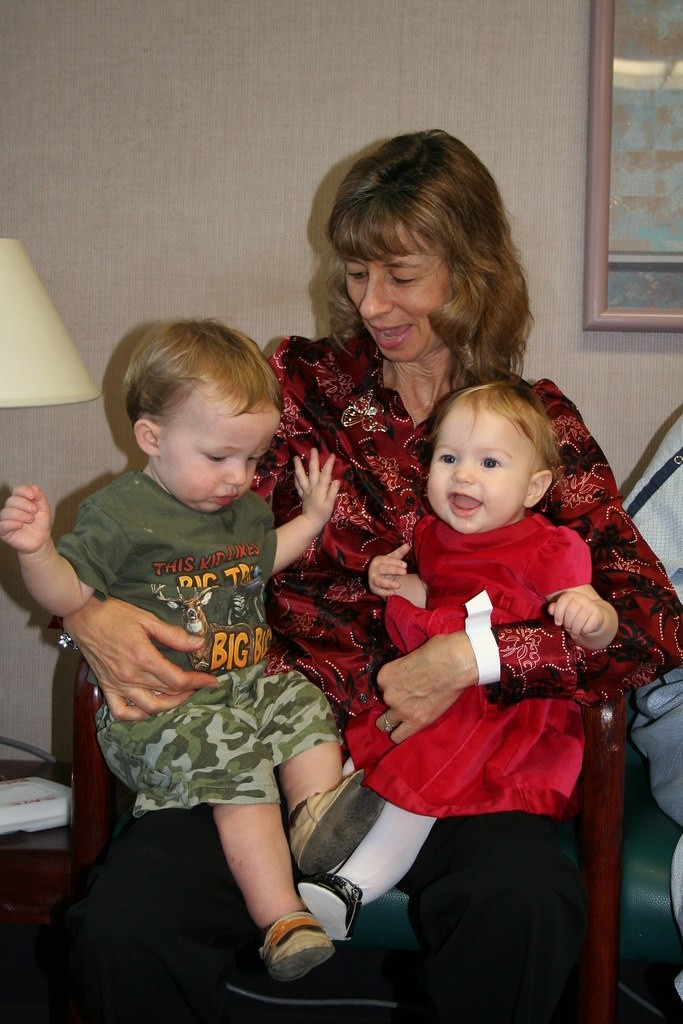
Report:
[0, 234, 100, 409]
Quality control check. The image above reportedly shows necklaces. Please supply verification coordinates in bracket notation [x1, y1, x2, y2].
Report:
[392, 371, 417, 425]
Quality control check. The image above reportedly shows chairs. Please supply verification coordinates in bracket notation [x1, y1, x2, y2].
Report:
[71, 655, 683, 1024]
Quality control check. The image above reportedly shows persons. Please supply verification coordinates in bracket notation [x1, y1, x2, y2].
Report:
[0, 129, 683, 981]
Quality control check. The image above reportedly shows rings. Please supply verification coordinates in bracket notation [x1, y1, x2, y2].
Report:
[382, 713, 396, 732]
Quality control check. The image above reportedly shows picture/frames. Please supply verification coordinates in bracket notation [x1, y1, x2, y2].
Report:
[581, 0, 683, 334]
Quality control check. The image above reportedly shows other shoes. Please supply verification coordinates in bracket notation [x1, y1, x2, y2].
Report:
[260, 911, 335, 981]
[287, 768, 385, 877]
[296, 876, 362, 942]
